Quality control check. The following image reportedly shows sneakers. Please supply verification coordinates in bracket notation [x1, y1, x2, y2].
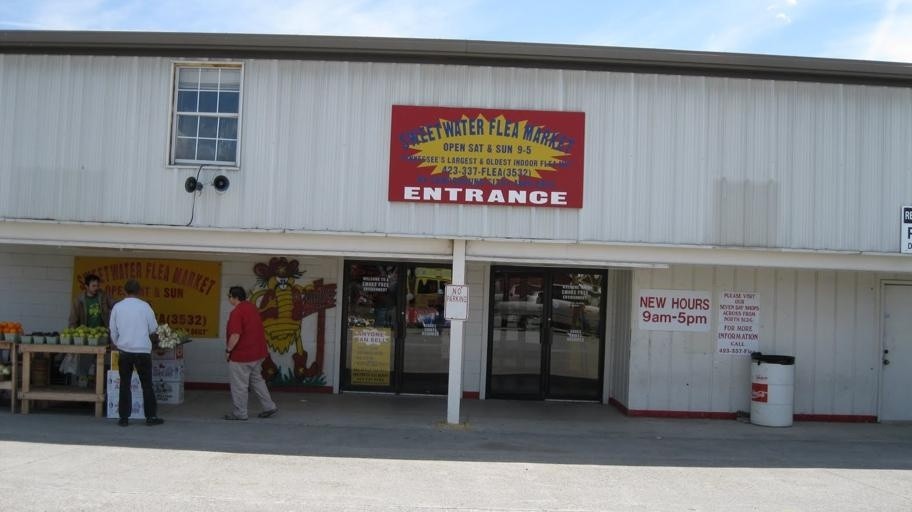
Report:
[259, 409, 279, 418]
[146, 417, 162, 425]
[225, 415, 248, 419]
[119, 418, 128, 426]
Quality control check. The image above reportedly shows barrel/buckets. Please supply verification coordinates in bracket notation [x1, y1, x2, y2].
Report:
[749, 351, 796, 427]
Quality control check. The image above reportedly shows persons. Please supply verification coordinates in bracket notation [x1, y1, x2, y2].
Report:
[223, 287, 279, 420]
[109, 280, 163, 426]
[67, 274, 113, 387]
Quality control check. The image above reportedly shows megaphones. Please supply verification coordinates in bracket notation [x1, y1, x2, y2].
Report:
[185, 177, 203, 193]
[213, 175, 230, 193]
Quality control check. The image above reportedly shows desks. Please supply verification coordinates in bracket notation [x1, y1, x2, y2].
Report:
[0, 340, 51, 414]
[18, 344, 107, 416]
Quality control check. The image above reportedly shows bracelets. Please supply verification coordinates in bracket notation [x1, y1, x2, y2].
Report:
[226, 348, 233, 353]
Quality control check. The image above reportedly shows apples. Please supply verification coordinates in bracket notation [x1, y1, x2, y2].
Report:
[61, 324, 109, 338]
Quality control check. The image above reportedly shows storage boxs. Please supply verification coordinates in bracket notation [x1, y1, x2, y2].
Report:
[107, 345, 184, 419]
